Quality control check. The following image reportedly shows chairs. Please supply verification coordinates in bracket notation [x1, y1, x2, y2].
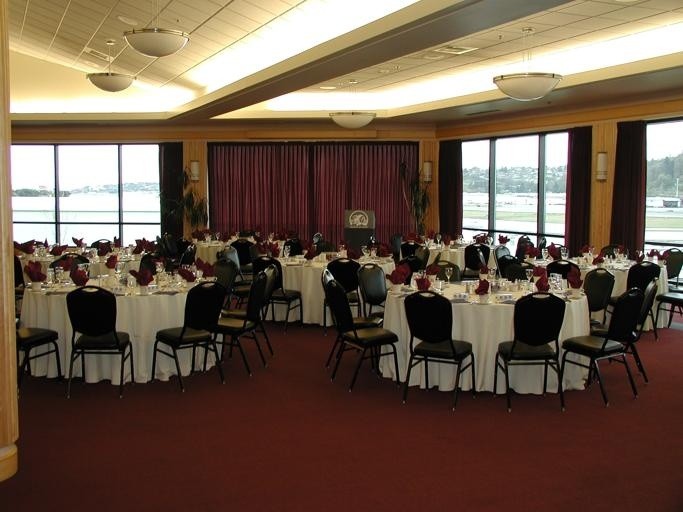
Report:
[214, 257, 265, 327]
[325, 280, 402, 393]
[491, 292, 566, 416]
[518, 241, 533, 262]
[255, 256, 303, 335]
[537, 238, 547, 259]
[499, 255, 528, 282]
[586, 269, 615, 325]
[217, 246, 260, 309]
[326, 258, 363, 326]
[493, 246, 511, 278]
[152, 281, 226, 391]
[655, 291, 683, 333]
[403, 292, 477, 415]
[547, 261, 580, 285]
[397, 256, 424, 284]
[426, 260, 460, 286]
[218, 271, 267, 378]
[658, 247, 683, 291]
[14, 318, 62, 395]
[598, 243, 635, 263]
[627, 261, 658, 341]
[560, 287, 639, 407]
[462, 246, 488, 279]
[469, 244, 490, 267]
[342, 228, 376, 266]
[592, 278, 658, 384]
[65, 284, 134, 399]
[13, 256, 26, 317]
[359, 263, 390, 326]
[401, 241, 429, 267]
[389, 233, 405, 262]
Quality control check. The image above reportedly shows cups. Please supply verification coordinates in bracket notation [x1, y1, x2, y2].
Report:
[557, 274, 562, 282]
[542, 248, 548, 261]
[392, 285, 401, 295]
[285, 246, 290, 252]
[550, 273, 557, 283]
[47, 269, 54, 284]
[458, 235, 462, 240]
[436, 235, 441, 245]
[120, 247, 123, 251]
[182, 265, 189, 270]
[371, 248, 377, 258]
[338, 245, 345, 252]
[339, 245, 344, 252]
[589, 248, 593, 255]
[56, 267, 64, 284]
[127, 279, 136, 293]
[613, 248, 619, 260]
[131, 255, 135, 261]
[548, 277, 556, 289]
[319, 254, 326, 262]
[561, 247, 566, 257]
[215, 232, 220, 241]
[363, 249, 370, 257]
[561, 253, 568, 261]
[526, 269, 533, 282]
[419, 270, 426, 276]
[561, 279, 567, 289]
[119, 253, 122, 259]
[445, 268, 452, 285]
[114, 263, 122, 279]
[270, 232, 274, 238]
[489, 269, 496, 279]
[284, 250, 289, 259]
[622, 260, 630, 268]
[424, 238, 429, 246]
[432, 282, 441, 292]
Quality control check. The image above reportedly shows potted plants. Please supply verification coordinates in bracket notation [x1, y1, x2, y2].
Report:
[401, 160, 433, 242]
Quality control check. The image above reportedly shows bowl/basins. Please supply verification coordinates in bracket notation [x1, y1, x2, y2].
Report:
[496, 294, 512, 302]
[453, 292, 468, 301]
[148, 285, 158, 292]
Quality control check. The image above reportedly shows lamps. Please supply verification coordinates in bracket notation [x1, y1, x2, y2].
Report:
[123, 1, 191, 58]
[189, 160, 202, 183]
[330, 82, 377, 130]
[596, 152, 609, 183]
[85, 42, 137, 94]
[493, 26, 562, 102]
[423, 161, 432, 186]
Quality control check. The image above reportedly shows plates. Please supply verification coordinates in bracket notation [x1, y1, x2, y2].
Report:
[286, 262, 304, 267]
